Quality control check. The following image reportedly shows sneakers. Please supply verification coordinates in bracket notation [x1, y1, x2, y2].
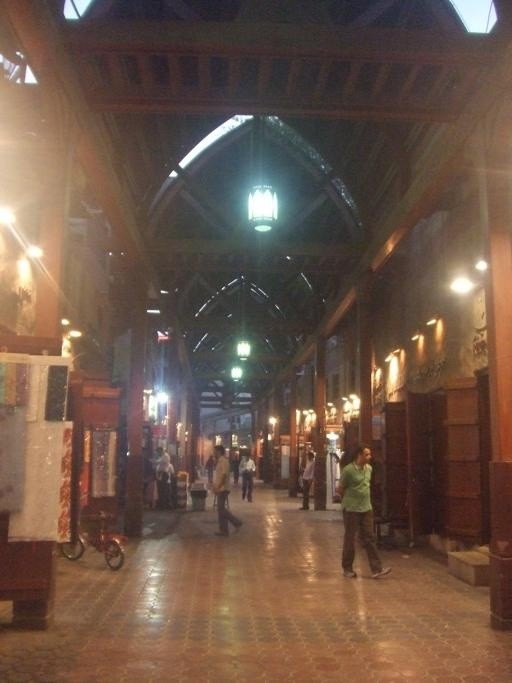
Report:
[344, 570, 356, 577]
[371, 567, 391, 578]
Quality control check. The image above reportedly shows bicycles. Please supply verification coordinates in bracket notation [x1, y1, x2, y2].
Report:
[60, 510, 126, 571]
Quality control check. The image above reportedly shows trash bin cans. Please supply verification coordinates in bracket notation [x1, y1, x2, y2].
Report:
[190, 483, 206, 511]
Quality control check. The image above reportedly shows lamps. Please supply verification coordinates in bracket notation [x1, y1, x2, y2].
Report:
[229, 287, 253, 384]
[246, 126, 280, 233]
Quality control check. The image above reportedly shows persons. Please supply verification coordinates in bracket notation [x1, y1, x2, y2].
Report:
[338, 443, 391, 577]
[206, 446, 241, 536]
[300, 451, 316, 510]
[152, 448, 176, 510]
[207, 447, 256, 502]
